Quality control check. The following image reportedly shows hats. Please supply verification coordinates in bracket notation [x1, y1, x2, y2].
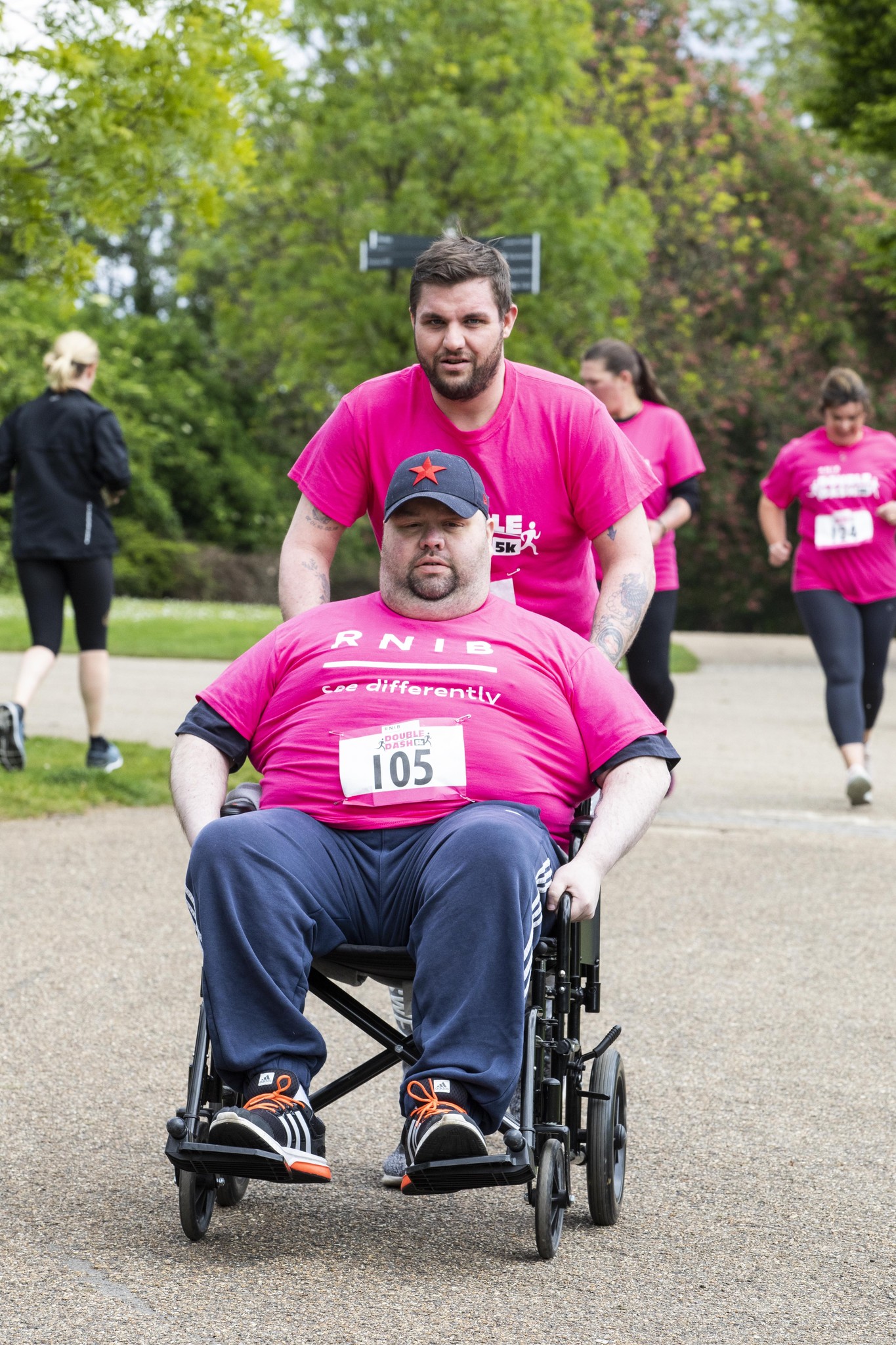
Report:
[383, 451, 489, 523]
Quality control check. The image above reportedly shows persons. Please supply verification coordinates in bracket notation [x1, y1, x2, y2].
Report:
[278, 242, 657, 1187]
[580, 341, 705, 797]
[169, 451, 679, 1194]
[0, 330, 132, 774]
[758, 368, 896, 806]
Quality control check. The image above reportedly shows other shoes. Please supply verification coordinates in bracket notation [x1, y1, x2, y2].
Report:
[847, 758, 874, 806]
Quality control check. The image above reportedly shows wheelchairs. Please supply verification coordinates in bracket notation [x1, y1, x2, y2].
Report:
[163, 781, 630, 1261]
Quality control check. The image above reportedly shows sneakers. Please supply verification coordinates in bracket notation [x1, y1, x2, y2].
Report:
[381, 1140, 407, 1183]
[86, 744, 124, 773]
[208, 1071, 331, 1183]
[400, 1078, 488, 1196]
[0, 701, 26, 771]
